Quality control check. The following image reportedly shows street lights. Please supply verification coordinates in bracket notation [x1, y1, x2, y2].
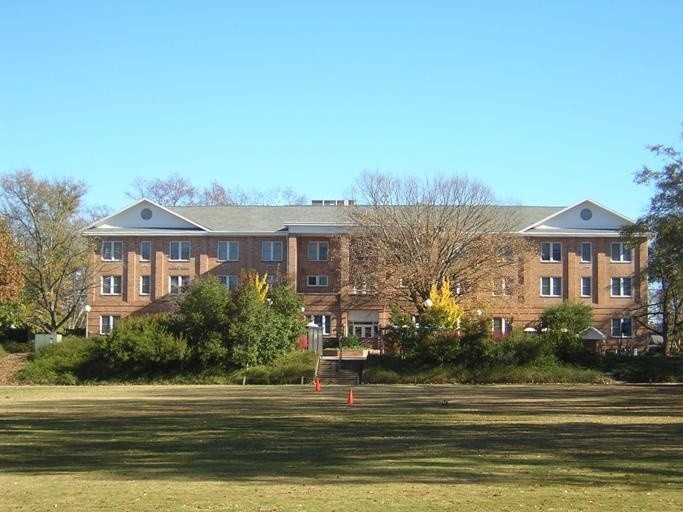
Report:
[83, 304, 93, 338]
[619, 316, 626, 356]
[300, 306, 308, 316]
[424, 299, 433, 310]
[477, 309, 483, 317]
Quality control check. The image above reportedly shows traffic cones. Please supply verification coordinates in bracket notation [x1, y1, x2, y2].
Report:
[312, 376, 322, 392]
[345, 386, 356, 405]
